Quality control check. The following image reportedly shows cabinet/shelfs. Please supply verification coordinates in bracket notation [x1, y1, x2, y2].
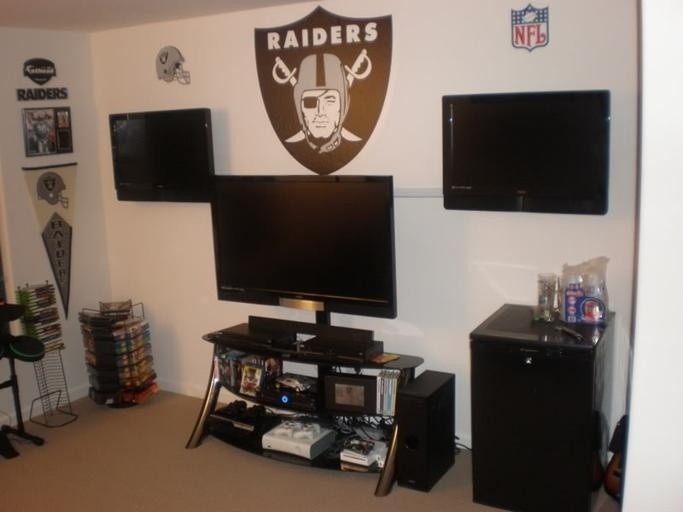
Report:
[184, 319, 424, 497]
[468, 304, 616, 512]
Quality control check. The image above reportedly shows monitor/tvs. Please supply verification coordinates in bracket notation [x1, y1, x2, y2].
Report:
[441, 89, 610, 215]
[207, 174, 397, 319]
[109, 107, 214, 202]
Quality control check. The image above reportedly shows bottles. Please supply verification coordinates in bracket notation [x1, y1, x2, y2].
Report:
[561, 273, 585, 324]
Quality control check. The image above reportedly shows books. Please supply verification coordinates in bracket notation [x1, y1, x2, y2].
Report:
[113, 318, 158, 402]
[375, 371, 397, 417]
[340, 439, 385, 466]
[16, 284, 64, 354]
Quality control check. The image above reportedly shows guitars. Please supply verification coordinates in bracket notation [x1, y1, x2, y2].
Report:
[604, 453, 623, 503]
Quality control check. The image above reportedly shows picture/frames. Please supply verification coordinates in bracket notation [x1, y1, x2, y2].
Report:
[321, 372, 377, 417]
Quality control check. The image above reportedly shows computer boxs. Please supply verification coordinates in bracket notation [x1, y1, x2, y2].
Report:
[394, 369, 456, 493]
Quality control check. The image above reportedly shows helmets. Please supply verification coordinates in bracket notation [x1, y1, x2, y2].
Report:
[154, 46, 185, 84]
